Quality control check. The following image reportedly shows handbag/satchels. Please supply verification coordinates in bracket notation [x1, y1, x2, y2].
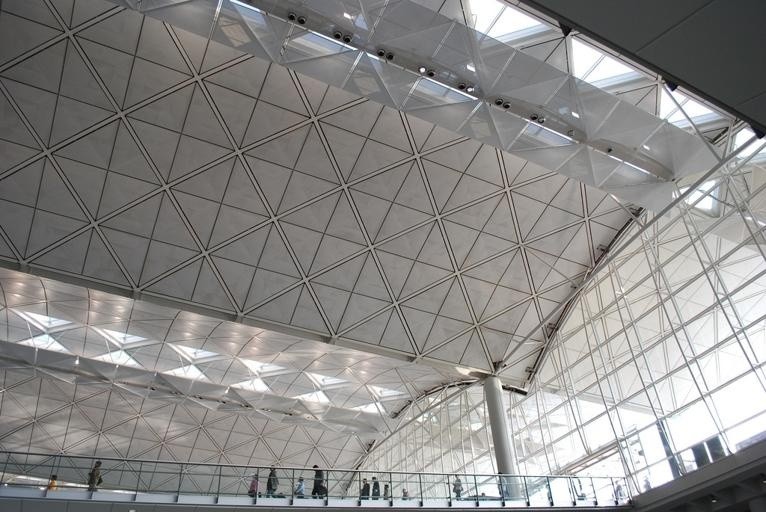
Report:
[317, 485, 327, 495]
[96, 476, 103, 486]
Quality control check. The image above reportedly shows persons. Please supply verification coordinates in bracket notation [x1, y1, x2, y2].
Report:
[248, 473, 262, 498]
[266, 464, 279, 498]
[88, 461, 103, 490]
[312, 465, 327, 499]
[294, 477, 305, 498]
[452, 474, 463, 500]
[47, 473, 57, 490]
[643, 478, 652, 490]
[497, 472, 508, 497]
[371, 476, 380, 500]
[402, 488, 410, 500]
[383, 484, 389, 500]
[361, 478, 370, 500]
[615, 480, 626, 500]
